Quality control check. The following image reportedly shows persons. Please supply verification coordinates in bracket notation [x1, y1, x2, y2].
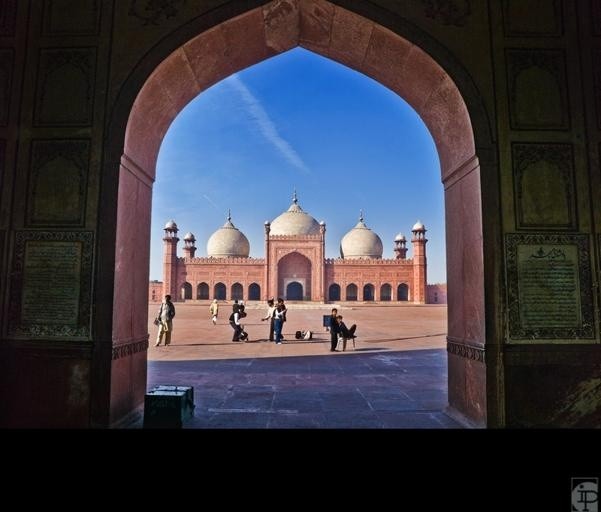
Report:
[261, 299, 285, 343]
[233, 299, 241, 312]
[326, 308, 341, 352]
[239, 301, 246, 312]
[273, 297, 288, 345]
[229, 311, 247, 342]
[335, 315, 358, 341]
[152, 294, 177, 347]
[209, 298, 219, 325]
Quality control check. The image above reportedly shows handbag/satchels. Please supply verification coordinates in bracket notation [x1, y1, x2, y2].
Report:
[153, 317, 159, 325]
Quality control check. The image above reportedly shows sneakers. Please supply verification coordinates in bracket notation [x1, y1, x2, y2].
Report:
[153, 344, 170, 346]
[277, 337, 284, 344]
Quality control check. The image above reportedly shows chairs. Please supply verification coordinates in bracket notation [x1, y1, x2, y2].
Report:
[335, 333, 356, 352]
